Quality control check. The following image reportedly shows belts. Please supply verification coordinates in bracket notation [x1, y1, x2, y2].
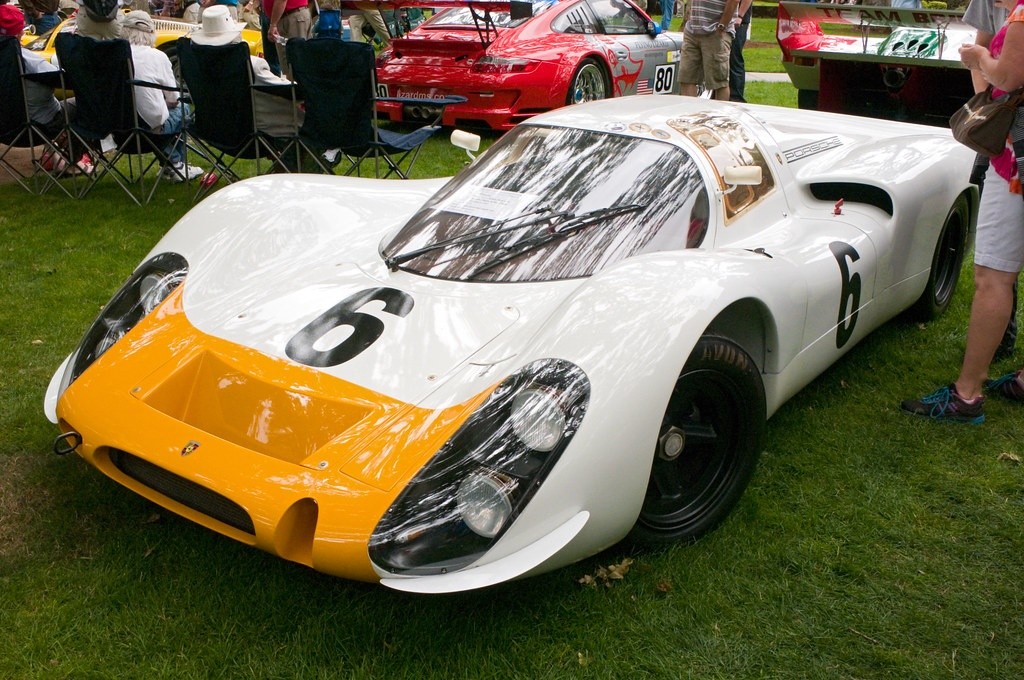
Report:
[280, 5, 309, 18]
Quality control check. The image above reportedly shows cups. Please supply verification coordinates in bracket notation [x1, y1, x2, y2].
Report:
[200, 172, 217, 189]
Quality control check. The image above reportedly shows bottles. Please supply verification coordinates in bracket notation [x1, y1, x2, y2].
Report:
[273, 33, 289, 46]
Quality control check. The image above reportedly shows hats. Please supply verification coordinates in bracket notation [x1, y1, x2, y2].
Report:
[190, 5, 247, 46]
[0, 4, 25, 34]
[82, 0, 119, 23]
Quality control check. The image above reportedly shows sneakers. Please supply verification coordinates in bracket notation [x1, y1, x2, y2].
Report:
[172, 163, 204, 180]
[67, 161, 93, 174]
[157, 166, 173, 179]
[900, 384, 984, 424]
[984, 373, 1024, 411]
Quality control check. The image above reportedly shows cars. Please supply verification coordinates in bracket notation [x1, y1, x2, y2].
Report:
[777, 0, 977, 92]
[374, 0, 715, 136]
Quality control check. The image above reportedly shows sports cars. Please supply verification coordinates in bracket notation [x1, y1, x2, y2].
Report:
[42, 92, 982, 595]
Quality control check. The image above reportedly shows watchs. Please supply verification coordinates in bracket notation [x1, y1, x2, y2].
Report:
[718, 24, 724, 29]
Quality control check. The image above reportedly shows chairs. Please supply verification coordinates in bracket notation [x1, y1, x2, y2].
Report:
[0, 33, 469, 208]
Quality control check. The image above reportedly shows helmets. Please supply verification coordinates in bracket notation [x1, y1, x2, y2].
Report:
[125, 10, 154, 33]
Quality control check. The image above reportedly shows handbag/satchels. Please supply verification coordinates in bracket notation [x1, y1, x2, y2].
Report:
[948, 81, 1024, 157]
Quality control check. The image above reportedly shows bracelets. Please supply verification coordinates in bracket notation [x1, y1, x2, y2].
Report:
[738, 15, 743, 19]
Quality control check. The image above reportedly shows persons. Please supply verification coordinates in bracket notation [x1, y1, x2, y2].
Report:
[901, 0, 1024, 424]
[729, 0, 753, 103]
[0, 0, 390, 181]
[658, 0, 675, 30]
[677, 0, 740, 101]
